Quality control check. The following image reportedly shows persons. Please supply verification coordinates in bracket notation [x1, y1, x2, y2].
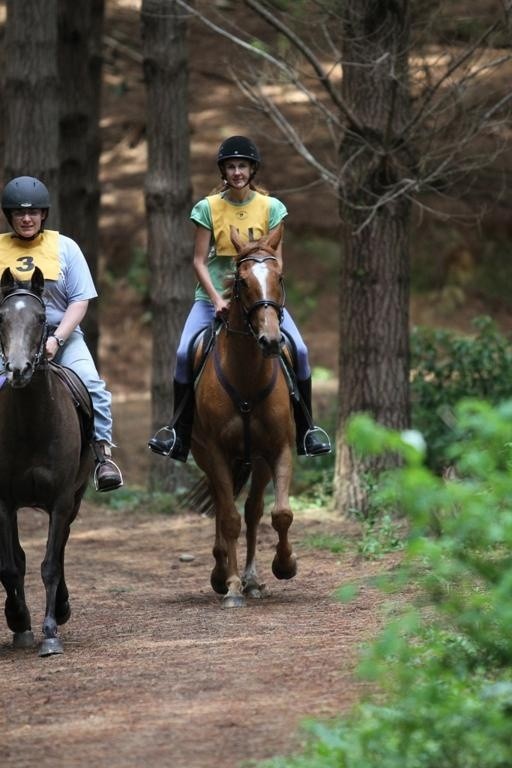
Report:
[147, 135, 333, 463]
[0, 173, 124, 493]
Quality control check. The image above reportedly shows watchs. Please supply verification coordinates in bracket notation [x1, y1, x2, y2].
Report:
[51, 331, 67, 351]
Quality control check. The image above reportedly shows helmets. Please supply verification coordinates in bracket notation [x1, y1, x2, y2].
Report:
[217, 135, 262, 165]
[1, 176, 52, 213]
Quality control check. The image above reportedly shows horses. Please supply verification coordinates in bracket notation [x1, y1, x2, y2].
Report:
[179, 220, 298, 613]
[0, 264, 99, 663]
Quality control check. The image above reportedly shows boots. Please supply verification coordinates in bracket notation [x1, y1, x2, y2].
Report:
[148, 378, 192, 463]
[95, 441, 120, 493]
[291, 375, 331, 456]
